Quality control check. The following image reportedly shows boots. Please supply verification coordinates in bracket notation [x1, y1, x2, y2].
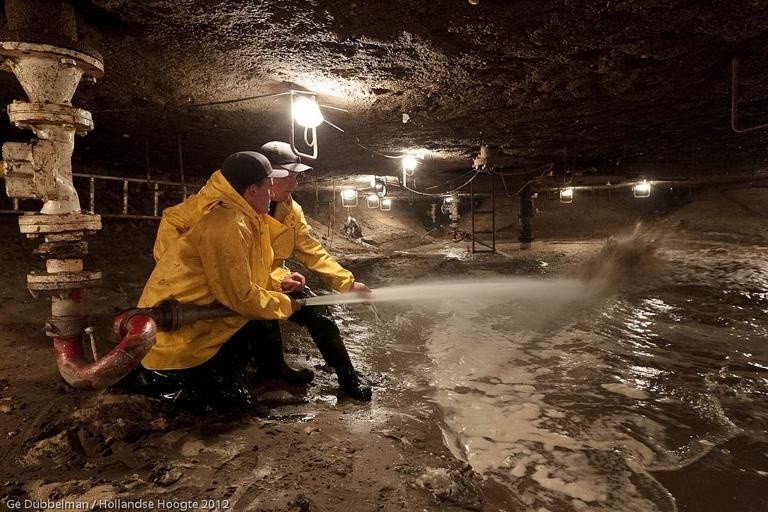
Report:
[336, 362, 371, 399]
[261, 346, 314, 387]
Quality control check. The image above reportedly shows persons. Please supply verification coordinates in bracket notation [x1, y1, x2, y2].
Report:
[129, 150, 315, 417]
[152, 137, 376, 401]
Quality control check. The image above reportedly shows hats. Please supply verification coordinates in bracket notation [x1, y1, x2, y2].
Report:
[259, 141, 314, 172]
[222, 152, 289, 181]
